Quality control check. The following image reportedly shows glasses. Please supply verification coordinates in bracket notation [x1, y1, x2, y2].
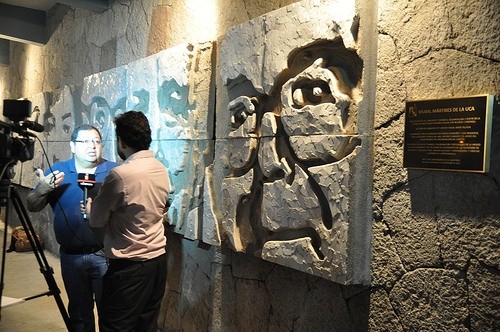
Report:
[74, 140, 101, 145]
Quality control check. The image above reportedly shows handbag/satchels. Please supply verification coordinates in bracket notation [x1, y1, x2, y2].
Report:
[5, 225, 45, 254]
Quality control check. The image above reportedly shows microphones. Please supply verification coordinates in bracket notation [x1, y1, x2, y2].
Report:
[77, 173, 97, 220]
[24, 119, 46, 133]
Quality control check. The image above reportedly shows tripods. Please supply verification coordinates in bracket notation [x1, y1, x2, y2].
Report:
[0, 184, 74, 332]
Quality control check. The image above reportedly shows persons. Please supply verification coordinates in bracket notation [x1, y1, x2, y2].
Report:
[79, 110, 170, 331]
[26, 124, 121, 332]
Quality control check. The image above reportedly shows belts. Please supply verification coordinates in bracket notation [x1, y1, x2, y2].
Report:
[60, 246, 104, 255]
